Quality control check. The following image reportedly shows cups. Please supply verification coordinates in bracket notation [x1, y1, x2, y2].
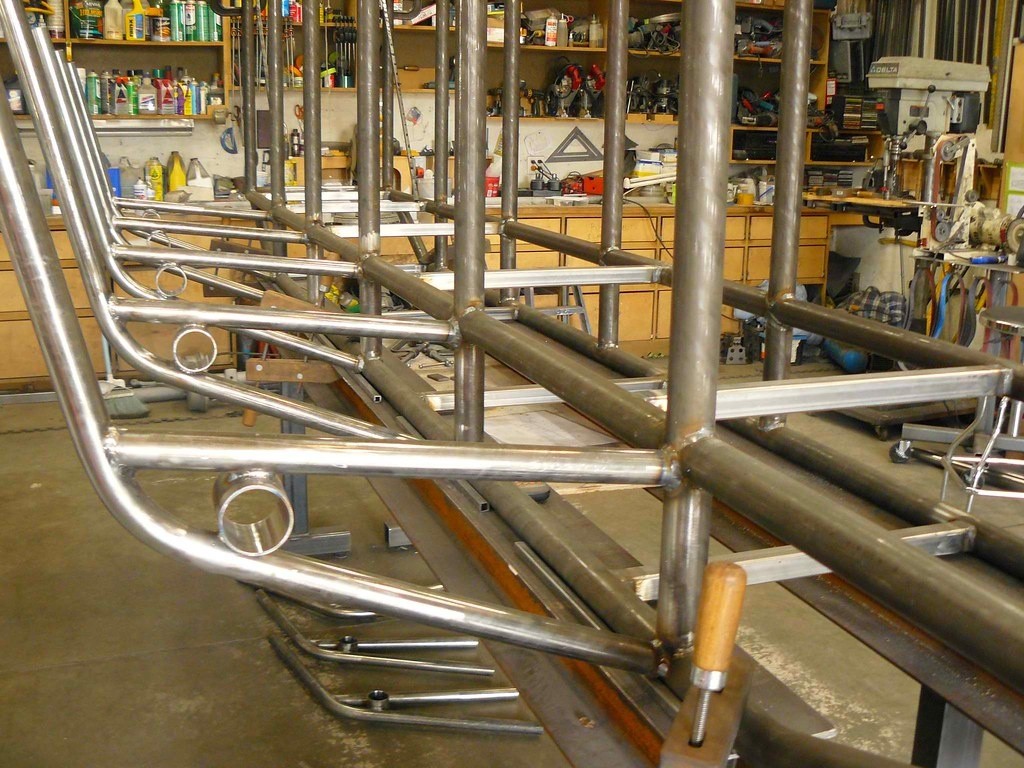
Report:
[485, 177, 499, 197]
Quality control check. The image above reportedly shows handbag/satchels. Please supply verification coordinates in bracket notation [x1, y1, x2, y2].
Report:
[836, 285, 909, 328]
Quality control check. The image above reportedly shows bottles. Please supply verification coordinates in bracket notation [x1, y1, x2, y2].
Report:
[737, 182, 754, 206]
[557, 13, 567, 47]
[24, 0, 222, 42]
[291, 128, 300, 157]
[4, 69, 27, 115]
[133, 150, 216, 202]
[338, 291, 359, 309]
[233, 0, 243, 20]
[545, 12, 558, 46]
[319, 2, 324, 24]
[75, 65, 225, 117]
[589, 14, 604, 48]
[281, 0, 302, 23]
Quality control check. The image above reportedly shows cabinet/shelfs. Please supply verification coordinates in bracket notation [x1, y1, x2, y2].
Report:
[1, 1, 883, 168]
[0, 209, 833, 391]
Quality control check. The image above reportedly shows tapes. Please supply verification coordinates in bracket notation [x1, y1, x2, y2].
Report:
[736, 192, 754, 205]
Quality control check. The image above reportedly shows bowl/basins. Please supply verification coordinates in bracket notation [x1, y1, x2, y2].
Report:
[417, 178, 451, 199]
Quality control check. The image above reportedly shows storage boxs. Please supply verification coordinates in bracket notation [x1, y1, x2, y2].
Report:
[288, 158, 458, 190]
[486, 17, 505, 44]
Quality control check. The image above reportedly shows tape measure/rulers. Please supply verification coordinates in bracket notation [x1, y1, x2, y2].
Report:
[544, 125, 605, 164]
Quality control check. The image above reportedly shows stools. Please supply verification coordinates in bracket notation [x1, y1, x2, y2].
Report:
[939, 305, 1023, 514]
